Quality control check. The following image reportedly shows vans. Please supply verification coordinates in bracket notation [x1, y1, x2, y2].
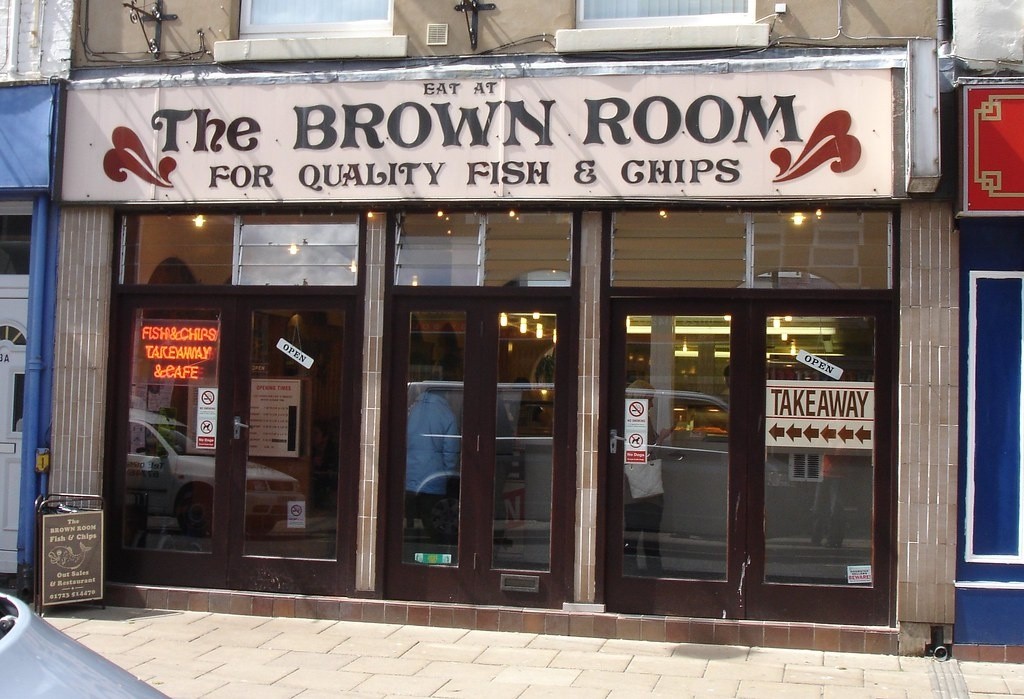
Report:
[405, 379, 798, 569]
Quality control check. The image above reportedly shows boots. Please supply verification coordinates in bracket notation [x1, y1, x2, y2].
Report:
[623, 556, 639, 575]
[646, 556, 672, 577]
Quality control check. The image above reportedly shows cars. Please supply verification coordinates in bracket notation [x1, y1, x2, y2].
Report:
[128, 410, 300, 539]
[0, 589, 174, 699]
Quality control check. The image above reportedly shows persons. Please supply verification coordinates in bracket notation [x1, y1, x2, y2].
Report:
[405, 387, 461, 544]
[811, 451, 856, 548]
[496, 389, 515, 546]
[723, 365, 731, 385]
[624, 380, 680, 577]
[512, 378, 554, 429]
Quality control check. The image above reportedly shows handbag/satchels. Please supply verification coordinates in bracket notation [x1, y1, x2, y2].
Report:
[624, 459, 664, 499]
